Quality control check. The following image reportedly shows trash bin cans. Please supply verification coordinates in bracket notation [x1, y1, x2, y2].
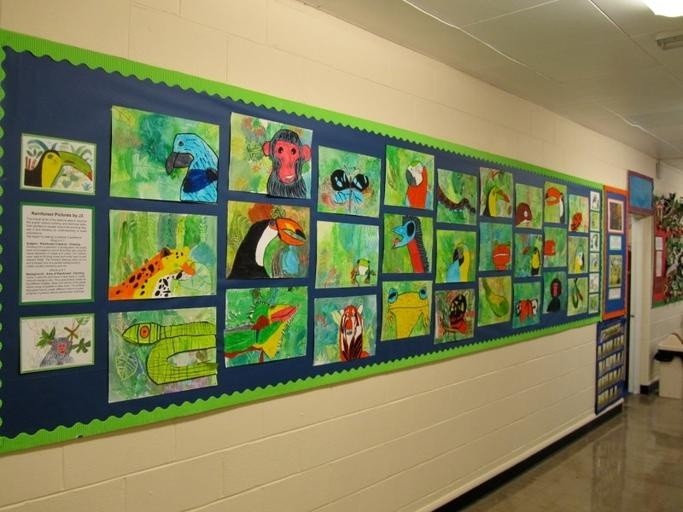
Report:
[657, 329, 683, 399]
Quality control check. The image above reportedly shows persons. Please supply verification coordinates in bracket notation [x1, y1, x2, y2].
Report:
[546, 278, 563, 312]
